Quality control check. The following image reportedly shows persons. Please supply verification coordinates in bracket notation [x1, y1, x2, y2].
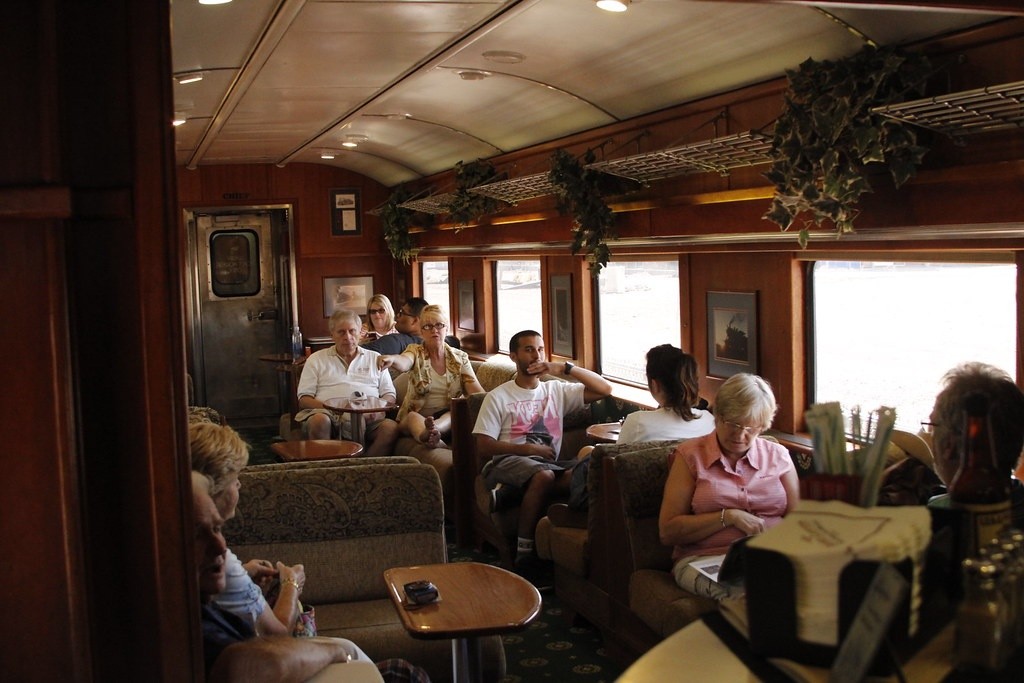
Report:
[189, 420, 306, 636]
[376, 304, 487, 449]
[295, 309, 399, 458]
[546, 344, 716, 528]
[188, 468, 432, 683]
[361, 297, 429, 355]
[658, 372, 801, 602]
[360, 293, 401, 345]
[928, 361, 1024, 523]
[470, 330, 610, 570]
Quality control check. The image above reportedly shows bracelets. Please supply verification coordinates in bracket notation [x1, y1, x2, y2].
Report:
[720, 508, 727, 529]
[281, 577, 298, 589]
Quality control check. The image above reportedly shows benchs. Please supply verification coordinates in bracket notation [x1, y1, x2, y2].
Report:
[392, 361, 595, 568]
[535, 440, 714, 668]
[223, 456, 507, 683]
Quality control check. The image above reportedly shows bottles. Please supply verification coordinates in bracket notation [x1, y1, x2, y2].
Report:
[947, 391, 1012, 614]
[292, 327, 303, 363]
[951, 526, 1024, 674]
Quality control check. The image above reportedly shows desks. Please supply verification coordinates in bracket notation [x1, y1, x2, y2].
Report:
[617, 602, 978, 683]
[384, 562, 541, 683]
[322, 396, 399, 443]
[272, 438, 364, 461]
[585, 422, 621, 442]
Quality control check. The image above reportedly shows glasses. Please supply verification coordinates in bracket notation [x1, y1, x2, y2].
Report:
[720, 416, 761, 436]
[400, 309, 421, 320]
[921, 418, 942, 433]
[370, 309, 385, 314]
[421, 323, 445, 330]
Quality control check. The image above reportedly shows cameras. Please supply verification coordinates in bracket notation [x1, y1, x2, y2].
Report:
[403, 580, 439, 605]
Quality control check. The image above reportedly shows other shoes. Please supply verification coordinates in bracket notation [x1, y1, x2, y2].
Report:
[514, 556, 553, 592]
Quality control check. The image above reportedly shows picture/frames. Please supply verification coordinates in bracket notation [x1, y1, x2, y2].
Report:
[550, 273, 574, 360]
[322, 274, 375, 319]
[704, 289, 760, 381]
[457, 279, 475, 333]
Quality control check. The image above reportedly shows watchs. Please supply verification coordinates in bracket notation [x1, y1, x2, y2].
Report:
[564, 362, 574, 374]
